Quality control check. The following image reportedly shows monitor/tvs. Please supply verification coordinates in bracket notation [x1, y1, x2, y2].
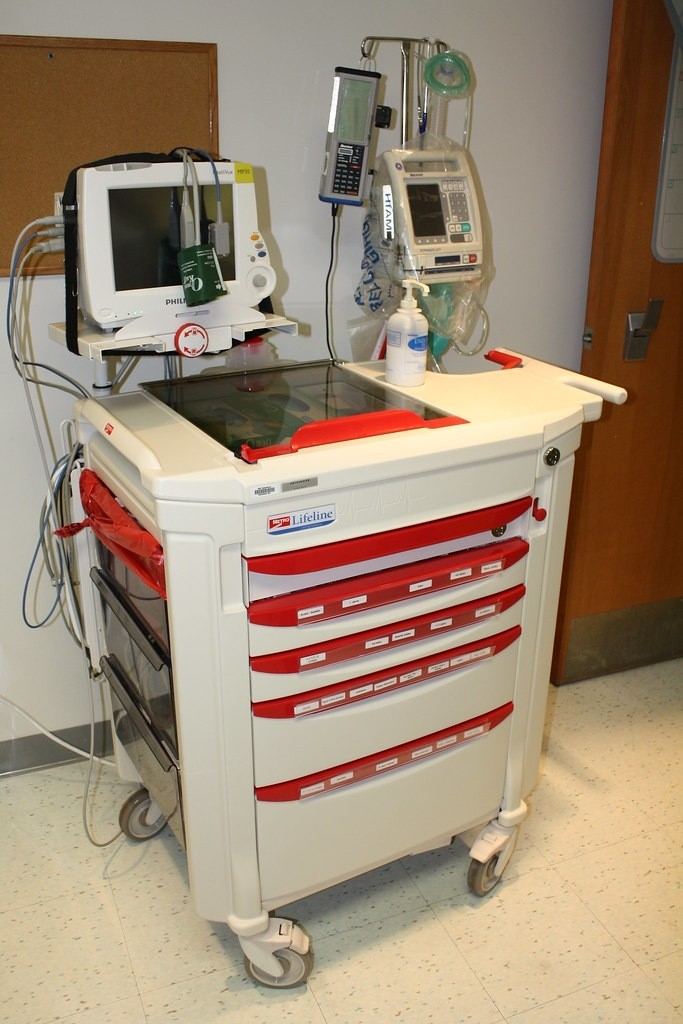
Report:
[72, 160, 277, 332]
